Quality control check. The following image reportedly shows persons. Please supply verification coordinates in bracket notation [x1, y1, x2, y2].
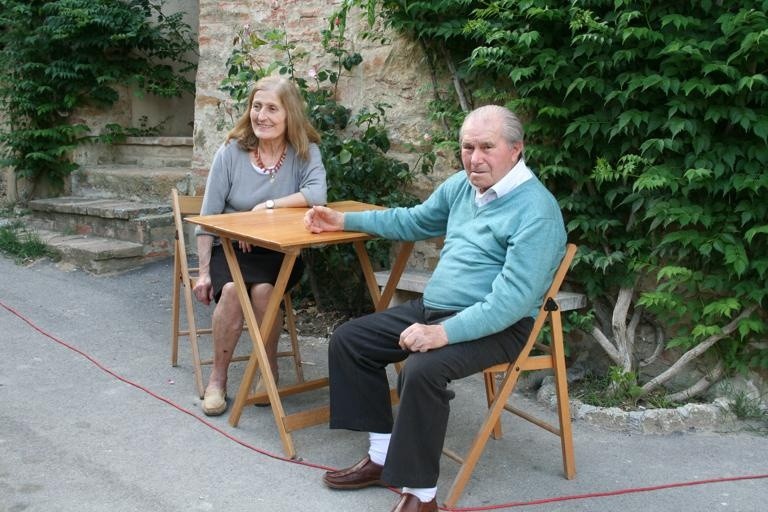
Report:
[300, 103, 568, 512]
[193, 75, 328, 417]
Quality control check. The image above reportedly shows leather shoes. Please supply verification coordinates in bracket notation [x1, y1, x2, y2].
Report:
[390, 492, 439, 512]
[322, 452, 385, 490]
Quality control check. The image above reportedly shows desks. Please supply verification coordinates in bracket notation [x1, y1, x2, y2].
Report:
[183, 197, 416, 455]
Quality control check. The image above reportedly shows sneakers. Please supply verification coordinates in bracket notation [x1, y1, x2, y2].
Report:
[200, 385, 229, 416]
[253, 373, 279, 407]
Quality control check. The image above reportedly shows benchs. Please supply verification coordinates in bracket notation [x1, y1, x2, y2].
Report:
[373, 267, 586, 308]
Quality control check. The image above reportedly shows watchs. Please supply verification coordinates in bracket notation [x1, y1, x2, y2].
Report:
[265, 199, 275, 209]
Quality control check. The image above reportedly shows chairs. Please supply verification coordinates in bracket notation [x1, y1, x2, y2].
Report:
[442, 242, 578, 510]
[171, 188, 304, 384]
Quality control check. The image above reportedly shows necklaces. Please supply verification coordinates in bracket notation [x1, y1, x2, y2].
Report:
[254, 142, 287, 176]
